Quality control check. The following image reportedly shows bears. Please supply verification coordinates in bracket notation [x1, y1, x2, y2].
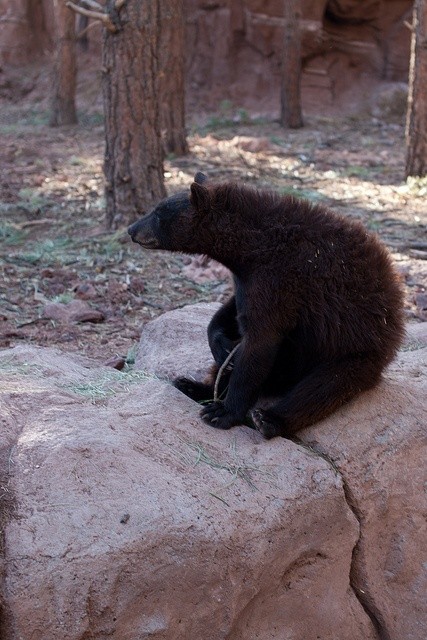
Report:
[125, 172, 407, 441]
